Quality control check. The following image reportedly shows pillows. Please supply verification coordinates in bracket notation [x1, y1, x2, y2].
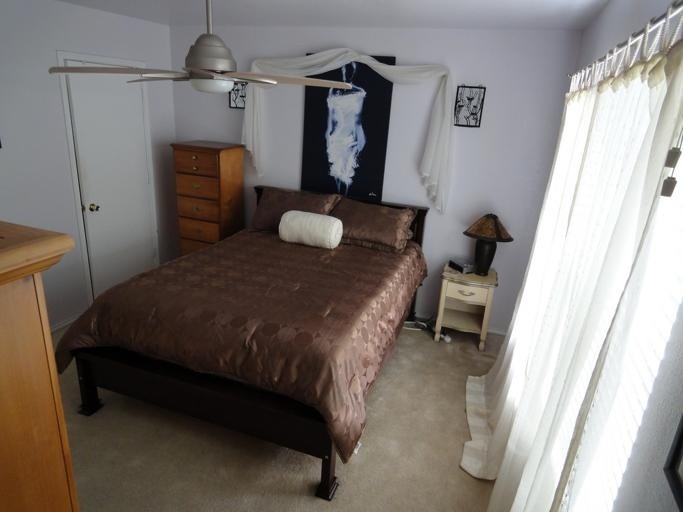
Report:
[252, 186, 419, 253]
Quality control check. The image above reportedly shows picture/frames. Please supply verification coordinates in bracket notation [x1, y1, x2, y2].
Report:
[228, 82, 247, 110]
[453, 85, 486, 128]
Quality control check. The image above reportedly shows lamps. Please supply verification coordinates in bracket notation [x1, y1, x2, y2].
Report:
[187, 78, 234, 94]
[462, 213, 514, 276]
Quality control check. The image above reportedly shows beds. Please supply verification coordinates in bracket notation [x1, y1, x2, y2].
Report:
[71, 185, 431, 502]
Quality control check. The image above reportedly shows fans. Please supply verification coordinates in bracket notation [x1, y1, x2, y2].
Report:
[48, 0, 352, 94]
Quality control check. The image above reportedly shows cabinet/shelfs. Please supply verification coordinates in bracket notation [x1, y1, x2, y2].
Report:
[169, 139, 245, 263]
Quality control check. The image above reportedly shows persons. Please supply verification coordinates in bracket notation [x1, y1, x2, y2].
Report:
[324, 62, 366, 196]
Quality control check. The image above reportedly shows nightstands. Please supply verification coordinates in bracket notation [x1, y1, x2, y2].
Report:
[431, 262, 499, 352]
